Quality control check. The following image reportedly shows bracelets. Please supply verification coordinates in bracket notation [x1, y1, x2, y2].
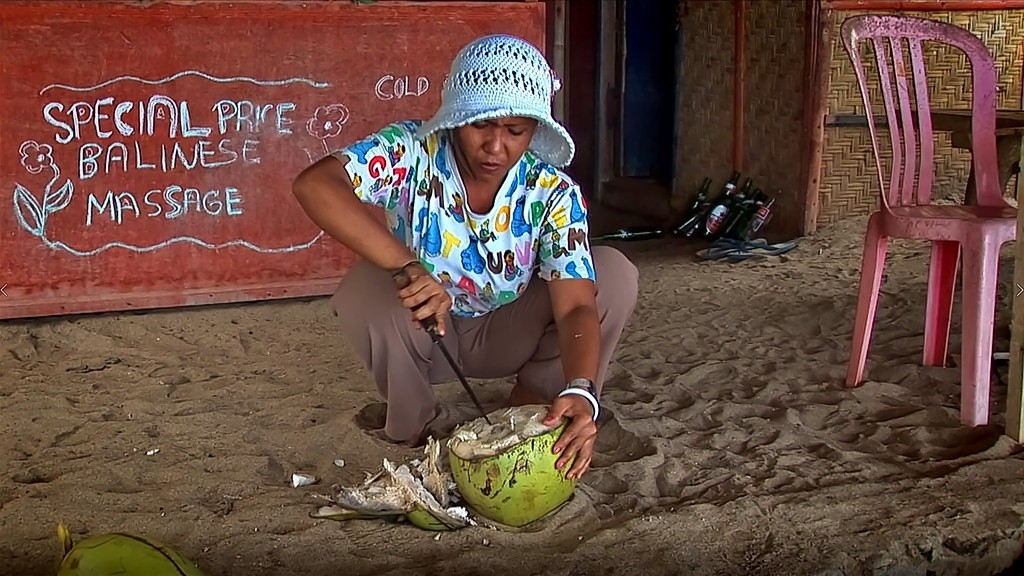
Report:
[557, 377, 599, 421]
[402, 261, 420, 268]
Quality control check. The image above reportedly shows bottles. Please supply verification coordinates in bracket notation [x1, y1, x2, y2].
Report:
[604, 229, 664, 241]
[680, 178, 713, 223]
[698, 171, 760, 240]
[674, 202, 717, 240]
[741, 190, 784, 242]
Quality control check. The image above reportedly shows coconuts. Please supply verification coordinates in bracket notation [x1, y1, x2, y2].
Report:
[54, 521, 208, 576]
[448, 405, 574, 525]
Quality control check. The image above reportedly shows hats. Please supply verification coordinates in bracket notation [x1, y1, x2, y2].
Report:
[416, 34, 575, 168]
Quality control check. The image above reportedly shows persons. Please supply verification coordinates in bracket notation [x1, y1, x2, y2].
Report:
[291, 33, 639, 479]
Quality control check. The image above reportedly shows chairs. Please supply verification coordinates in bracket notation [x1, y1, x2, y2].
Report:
[841, 16, 1020, 428]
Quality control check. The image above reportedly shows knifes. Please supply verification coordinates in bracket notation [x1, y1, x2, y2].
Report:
[392, 270, 492, 426]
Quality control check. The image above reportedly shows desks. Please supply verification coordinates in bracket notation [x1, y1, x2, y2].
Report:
[891, 109, 1024, 444]
